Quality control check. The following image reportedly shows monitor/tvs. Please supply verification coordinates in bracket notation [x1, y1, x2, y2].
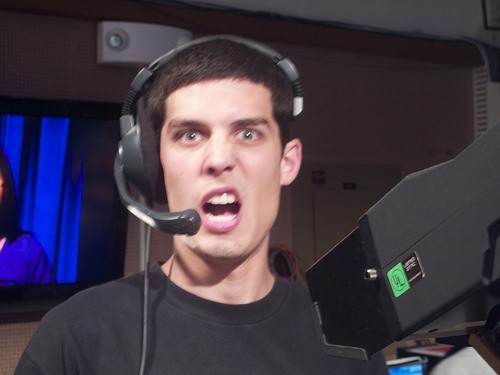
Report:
[0, 94, 129, 297]
[386, 357, 424, 375]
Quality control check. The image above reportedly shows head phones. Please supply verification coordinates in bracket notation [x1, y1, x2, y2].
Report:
[118, 34, 303, 203]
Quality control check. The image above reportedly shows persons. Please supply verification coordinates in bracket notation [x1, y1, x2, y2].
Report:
[13, 39, 392, 375]
[0, 149, 56, 285]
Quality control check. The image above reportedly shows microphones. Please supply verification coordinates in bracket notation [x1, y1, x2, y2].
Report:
[114, 153, 202, 235]
